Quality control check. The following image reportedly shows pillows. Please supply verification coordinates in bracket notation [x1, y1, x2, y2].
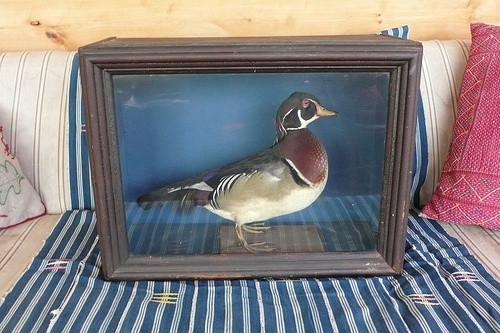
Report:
[418, 22, 500, 230]
[0, 125, 46, 229]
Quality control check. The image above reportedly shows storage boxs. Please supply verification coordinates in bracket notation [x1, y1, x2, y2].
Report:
[78, 35, 423, 282]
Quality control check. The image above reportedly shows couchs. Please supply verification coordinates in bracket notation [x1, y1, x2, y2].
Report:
[2, 39, 500, 333]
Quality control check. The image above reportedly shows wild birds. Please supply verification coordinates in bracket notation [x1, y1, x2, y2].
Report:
[138, 92, 338, 254]
